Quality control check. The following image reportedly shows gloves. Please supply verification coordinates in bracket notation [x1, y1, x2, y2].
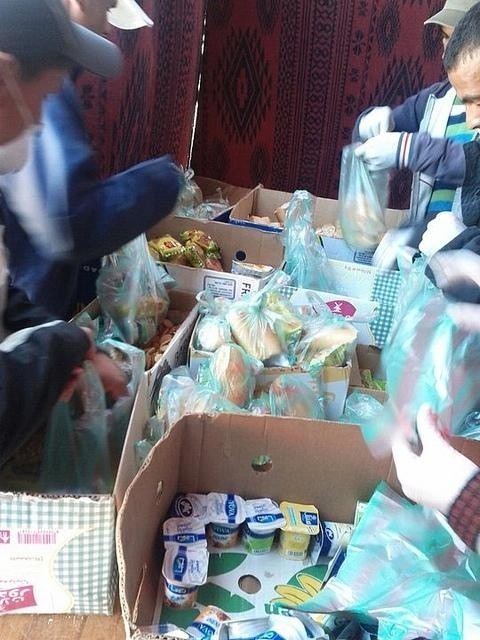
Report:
[358, 107, 394, 140]
[417, 210, 468, 256]
[354, 133, 399, 172]
[390, 404, 480, 515]
[438, 251, 480, 327]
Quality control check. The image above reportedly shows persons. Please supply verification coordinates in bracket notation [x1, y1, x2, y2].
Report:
[349, 0, 479, 231]
[388, 402, 480, 551]
[1, 0, 122, 472]
[367, 4, 479, 309]
[0, 0, 185, 308]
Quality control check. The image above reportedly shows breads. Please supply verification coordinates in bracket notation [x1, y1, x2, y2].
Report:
[199, 308, 355, 419]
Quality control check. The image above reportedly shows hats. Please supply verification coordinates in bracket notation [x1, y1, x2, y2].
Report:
[0, 0, 121, 79]
[107, 0, 153, 30]
[422, 1, 480, 30]
